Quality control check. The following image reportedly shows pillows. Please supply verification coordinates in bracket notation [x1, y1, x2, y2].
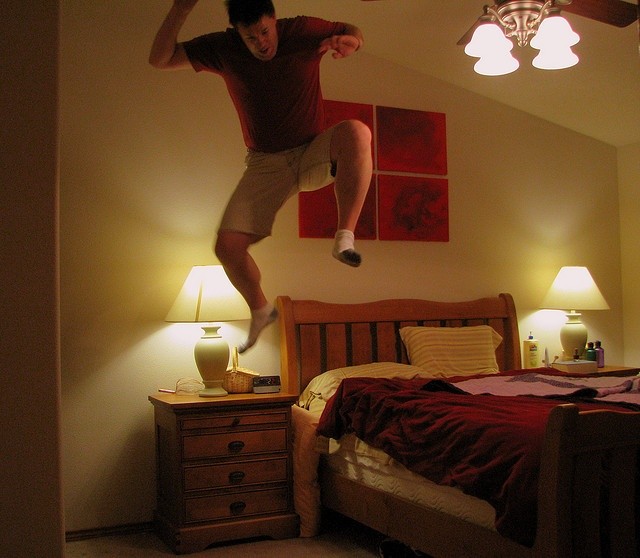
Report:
[299, 362, 437, 410]
[398, 325, 503, 378]
[290, 404, 322, 538]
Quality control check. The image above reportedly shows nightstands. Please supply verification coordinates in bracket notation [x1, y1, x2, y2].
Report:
[148, 392, 300, 555]
[596, 365, 640, 377]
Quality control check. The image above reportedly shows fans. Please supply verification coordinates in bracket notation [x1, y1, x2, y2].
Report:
[459, 2, 639, 46]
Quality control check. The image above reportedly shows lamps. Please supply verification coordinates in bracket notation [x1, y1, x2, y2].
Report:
[164, 265, 252, 397]
[457, 0, 581, 76]
[539, 266, 610, 360]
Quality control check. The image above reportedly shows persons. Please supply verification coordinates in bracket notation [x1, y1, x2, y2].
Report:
[148, 0, 373, 354]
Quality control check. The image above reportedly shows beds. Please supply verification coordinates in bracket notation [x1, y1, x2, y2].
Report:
[277, 293, 639, 557]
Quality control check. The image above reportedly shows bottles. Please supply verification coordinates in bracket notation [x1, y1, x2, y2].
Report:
[573, 348, 579, 362]
[595, 341, 604, 368]
[585, 342, 596, 361]
